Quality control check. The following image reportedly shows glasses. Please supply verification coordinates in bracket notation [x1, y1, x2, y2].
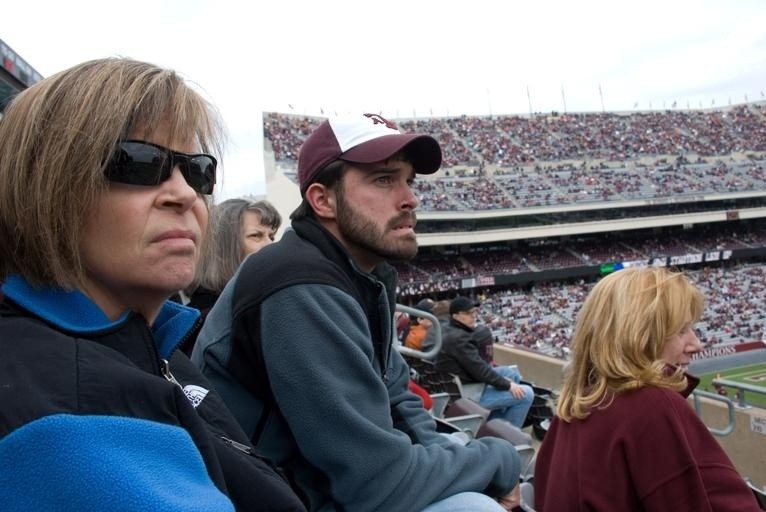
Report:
[100, 139, 217, 196]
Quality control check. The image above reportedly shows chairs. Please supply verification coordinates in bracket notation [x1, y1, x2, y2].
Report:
[430, 397, 537, 511]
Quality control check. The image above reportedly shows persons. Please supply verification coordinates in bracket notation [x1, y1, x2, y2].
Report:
[534, 263, 764, 512]
[185, 107, 529, 512]
[432, 294, 535, 435]
[258, 110, 326, 176]
[718, 386, 728, 398]
[713, 371, 721, 394]
[0, 52, 313, 512]
[172, 193, 280, 365]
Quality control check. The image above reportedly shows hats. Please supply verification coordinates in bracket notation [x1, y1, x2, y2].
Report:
[298, 109, 444, 187]
[415, 298, 481, 315]
